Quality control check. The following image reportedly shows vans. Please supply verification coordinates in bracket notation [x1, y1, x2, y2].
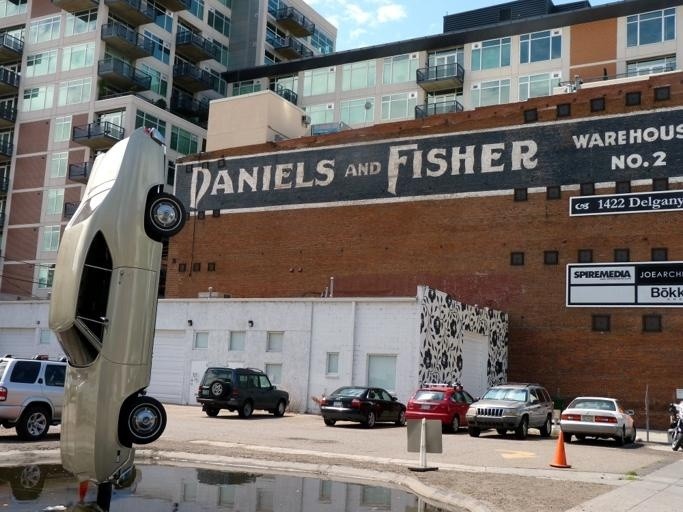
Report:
[0, 354, 67, 441]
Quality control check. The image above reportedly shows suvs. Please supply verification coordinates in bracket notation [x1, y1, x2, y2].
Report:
[195, 366, 291, 420]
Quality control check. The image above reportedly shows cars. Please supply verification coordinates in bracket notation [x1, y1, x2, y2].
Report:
[559, 395, 636, 447]
[48, 125, 187, 489]
[319, 386, 406, 428]
[465, 382, 555, 440]
[403, 382, 476, 433]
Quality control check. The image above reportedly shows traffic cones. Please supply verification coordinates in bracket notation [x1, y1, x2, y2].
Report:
[549, 431, 571, 468]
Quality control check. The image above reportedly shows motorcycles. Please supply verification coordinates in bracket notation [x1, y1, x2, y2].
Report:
[667, 399, 682, 451]
